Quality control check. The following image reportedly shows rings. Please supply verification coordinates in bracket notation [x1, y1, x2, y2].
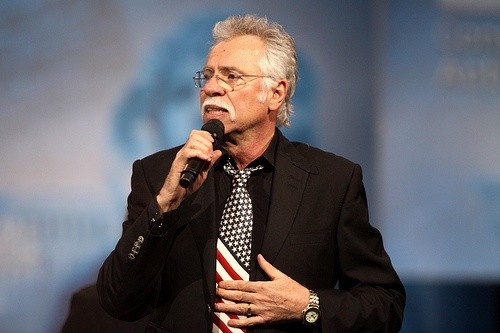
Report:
[247, 304, 252, 316]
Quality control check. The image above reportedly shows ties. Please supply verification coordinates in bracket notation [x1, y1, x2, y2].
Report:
[211, 159, 265, 333]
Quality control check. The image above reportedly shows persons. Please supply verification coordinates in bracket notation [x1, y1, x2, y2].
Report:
[96, 14, 405, 333]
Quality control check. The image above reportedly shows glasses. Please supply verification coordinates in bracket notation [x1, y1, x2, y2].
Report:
[193, 70, 272, 88]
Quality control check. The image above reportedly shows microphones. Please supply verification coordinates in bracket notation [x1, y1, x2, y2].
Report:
[178, 118, 224, 188]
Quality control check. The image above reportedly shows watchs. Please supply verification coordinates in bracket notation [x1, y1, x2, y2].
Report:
[302, 289, 321, 326]
